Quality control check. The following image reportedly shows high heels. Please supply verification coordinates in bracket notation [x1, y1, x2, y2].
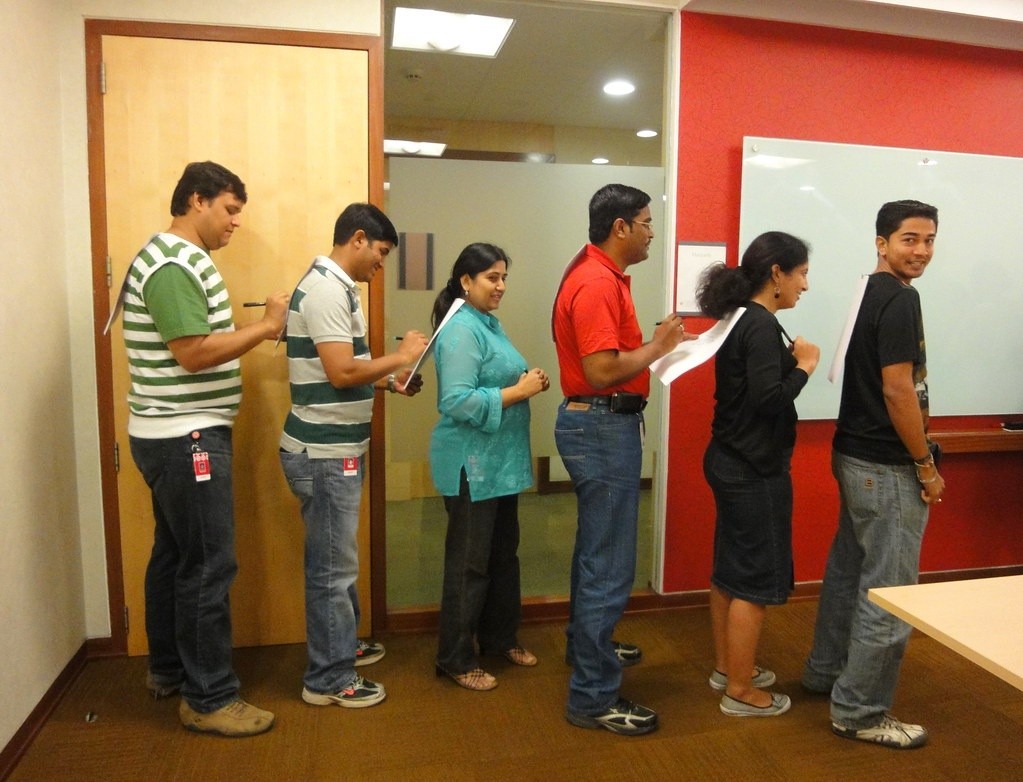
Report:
[479, 641, 538, 665]
[435, 656, 497, 690]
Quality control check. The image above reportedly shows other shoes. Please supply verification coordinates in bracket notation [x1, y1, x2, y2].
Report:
[708, 662, 776, 690]
[720, 691, 791, 716]
[832, 709, 929, 748]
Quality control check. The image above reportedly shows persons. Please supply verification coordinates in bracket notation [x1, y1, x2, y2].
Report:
[554, 184, 698, 737]
[427, 243, 550, 691]
[126, 161, 291, 738]
[803, 199, 947, 749]
[693, 231, 822, 718]
[279, 202, 430, 708]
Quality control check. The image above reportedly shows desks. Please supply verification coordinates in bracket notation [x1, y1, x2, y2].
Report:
[868, 575, 1023, 694]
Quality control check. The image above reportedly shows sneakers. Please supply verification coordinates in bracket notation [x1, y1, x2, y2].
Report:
[610, 640, 642, 665]
[179, 693, 275, 736]
[566, 695, 657, 735]
[352, 641, 386, 667]
[144, 671, 183, 694]
[301, 671, 385, 708]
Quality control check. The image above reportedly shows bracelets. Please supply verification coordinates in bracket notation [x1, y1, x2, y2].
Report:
[913, 454, 934, 467]
[388, 375, 394, 390]
[916, 465, 938, 484]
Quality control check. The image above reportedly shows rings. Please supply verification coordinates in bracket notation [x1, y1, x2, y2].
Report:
[937, 498, 941, 503]
[679, 324, 684, 331]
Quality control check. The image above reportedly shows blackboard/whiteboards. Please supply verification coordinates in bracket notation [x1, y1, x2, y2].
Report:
[737, 135, 1023, 421]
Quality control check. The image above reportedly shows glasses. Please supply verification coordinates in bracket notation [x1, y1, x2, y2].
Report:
[617, 218, 653, 234]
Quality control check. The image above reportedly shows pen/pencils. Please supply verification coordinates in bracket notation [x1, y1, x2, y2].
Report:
[778, 325, 794, 344]
[393, 336, 404, 341]
[655, 321, 662, 326]
[243, 302, 266, 307]
[523, 368, 528, 374]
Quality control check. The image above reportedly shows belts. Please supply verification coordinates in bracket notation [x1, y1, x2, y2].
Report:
[568, 396, 645, 442]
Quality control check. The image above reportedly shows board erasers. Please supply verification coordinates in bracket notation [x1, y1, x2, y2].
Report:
[1002, 422, 1023, 433]
